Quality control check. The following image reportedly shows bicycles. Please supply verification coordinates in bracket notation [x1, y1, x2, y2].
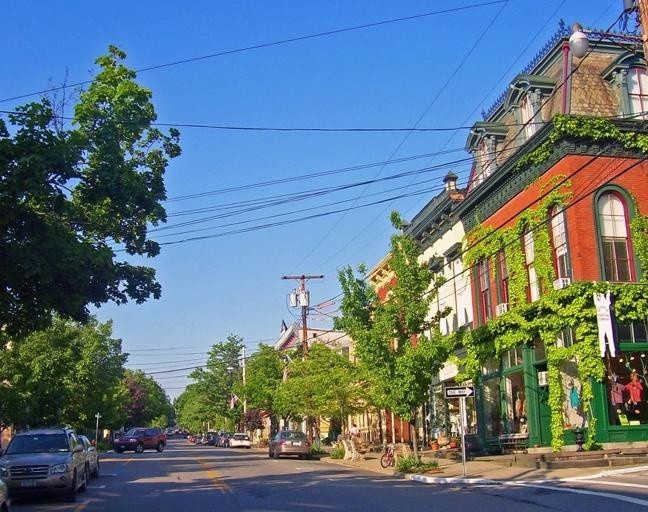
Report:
[380, 443, 393, 467]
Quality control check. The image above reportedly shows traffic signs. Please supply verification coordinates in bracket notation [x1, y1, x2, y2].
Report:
[444, 385, 476, 399]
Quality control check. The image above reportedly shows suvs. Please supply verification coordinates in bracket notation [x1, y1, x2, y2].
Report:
[0, 427, 87, 501]
[113, 425, 167, 453]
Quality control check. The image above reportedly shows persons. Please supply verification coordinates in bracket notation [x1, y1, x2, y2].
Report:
[626, 372, 643, 421]
[610, 374, 625, 425]
[515, 391, 522, 432]
[351, 424, 359, 442]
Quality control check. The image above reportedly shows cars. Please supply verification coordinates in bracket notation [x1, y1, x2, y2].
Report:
[168, 425, 231, 447]
[75, 434, 100, 487]
[228, 433, 252, 448]
[268, 430, 312, 460]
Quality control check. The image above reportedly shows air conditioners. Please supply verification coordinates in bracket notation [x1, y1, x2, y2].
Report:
[495, 303, 507, 317]
[538, 372, 548, 386]
[553, 278, 571, 290]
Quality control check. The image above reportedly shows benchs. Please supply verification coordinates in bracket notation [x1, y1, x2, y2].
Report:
[487, 432, 530, 454]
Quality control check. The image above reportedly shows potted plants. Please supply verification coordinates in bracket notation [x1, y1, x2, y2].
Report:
[429, 438, 439, 450]
[450, 437, 457, 448]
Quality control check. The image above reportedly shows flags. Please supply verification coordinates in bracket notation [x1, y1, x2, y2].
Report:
[230, 393, 238, 409]
[282, 356, 291, 381]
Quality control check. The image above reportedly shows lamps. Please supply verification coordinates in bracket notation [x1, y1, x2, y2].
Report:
[568, 22, 648, 58]
